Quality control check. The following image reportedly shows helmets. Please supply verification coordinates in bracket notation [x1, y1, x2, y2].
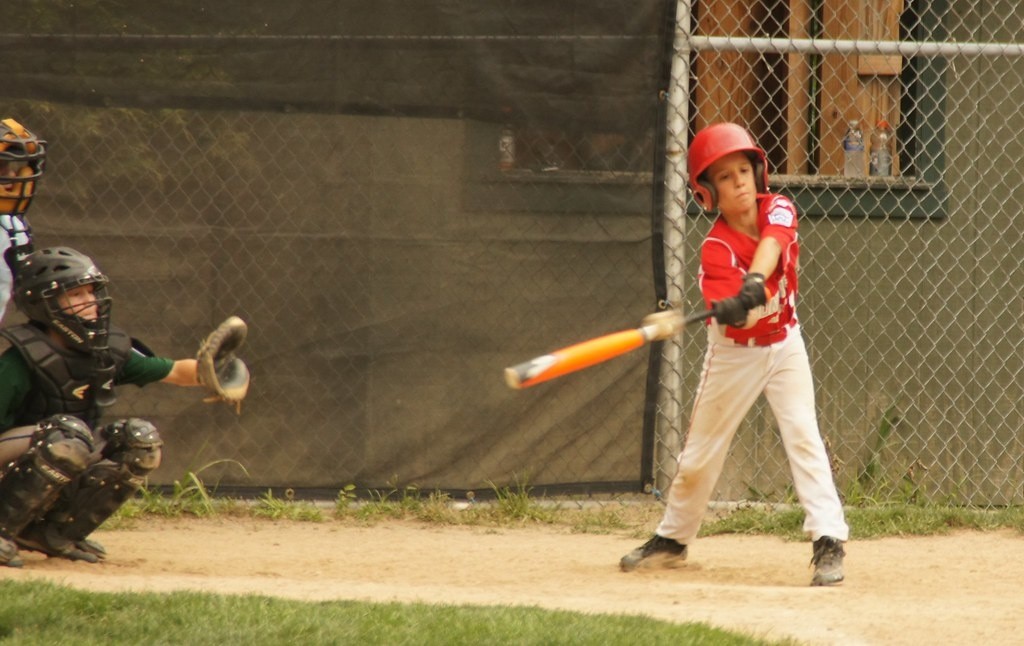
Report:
[15, 248, 112, 355]
[690, 123, 770, 211]
[0, 119, 45, 218]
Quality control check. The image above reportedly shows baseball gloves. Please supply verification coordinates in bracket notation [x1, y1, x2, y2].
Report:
[196, 316, 251, 403]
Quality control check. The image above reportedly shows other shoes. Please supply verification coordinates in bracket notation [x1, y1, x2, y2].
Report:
[16, 534, 107, 563]
[0, 537, 47, 567]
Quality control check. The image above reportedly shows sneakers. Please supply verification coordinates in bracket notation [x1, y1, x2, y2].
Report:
[619, 534, 687, 571]
[809, 536, 845, 586]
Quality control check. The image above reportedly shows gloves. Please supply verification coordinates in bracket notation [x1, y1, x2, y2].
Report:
[738, 274, 767, 308]
[711, 298, 747, 327]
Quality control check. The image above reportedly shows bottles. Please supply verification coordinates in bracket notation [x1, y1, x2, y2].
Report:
[843, 120, 865, 176]
[870, 123, 892, 177]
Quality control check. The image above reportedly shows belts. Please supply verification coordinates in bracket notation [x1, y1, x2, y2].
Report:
[732, 329, 787, 346]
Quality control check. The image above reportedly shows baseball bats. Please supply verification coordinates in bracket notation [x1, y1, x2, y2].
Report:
[503, 282, 772, 391]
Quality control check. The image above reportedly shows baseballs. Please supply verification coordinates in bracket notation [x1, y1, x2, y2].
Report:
[644, 310, 684, 340]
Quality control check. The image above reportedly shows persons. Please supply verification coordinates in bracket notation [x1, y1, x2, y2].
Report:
[0, 246, 227, 567]
[620, 123, 851, 586]
[0, 118, 48, 325]
